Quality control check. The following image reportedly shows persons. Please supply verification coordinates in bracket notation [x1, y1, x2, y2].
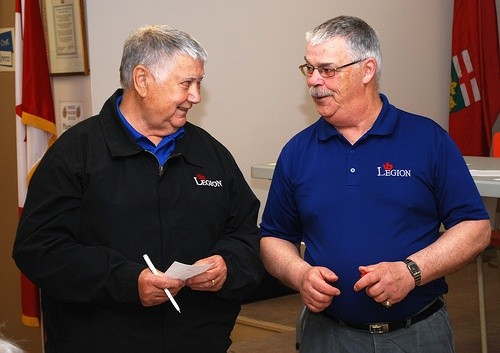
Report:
[258, 15, 492, 353]
[12, 24, 262, 353]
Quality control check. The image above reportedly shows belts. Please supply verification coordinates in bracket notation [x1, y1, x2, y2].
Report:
[322, 299, 444, 334]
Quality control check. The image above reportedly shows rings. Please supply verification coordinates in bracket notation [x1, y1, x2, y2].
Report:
[384, 301, 391, 308]
[212, 279, 215, 286]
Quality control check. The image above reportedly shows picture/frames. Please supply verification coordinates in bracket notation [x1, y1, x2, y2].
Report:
[40, 0, 89, 76]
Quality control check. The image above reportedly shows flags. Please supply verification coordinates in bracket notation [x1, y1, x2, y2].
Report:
[448, 0, 500, 249]
[14, 0, 56, 329]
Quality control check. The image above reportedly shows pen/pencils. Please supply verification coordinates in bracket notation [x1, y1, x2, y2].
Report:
[143, 254, 181, 314]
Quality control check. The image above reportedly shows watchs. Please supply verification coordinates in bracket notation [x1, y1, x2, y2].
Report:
[403, 258, 421, 289]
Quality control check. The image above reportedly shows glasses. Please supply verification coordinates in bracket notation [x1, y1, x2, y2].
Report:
[299, 56, 369, 78]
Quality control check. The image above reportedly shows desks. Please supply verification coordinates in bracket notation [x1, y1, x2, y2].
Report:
[251, 156, 500, 353]
